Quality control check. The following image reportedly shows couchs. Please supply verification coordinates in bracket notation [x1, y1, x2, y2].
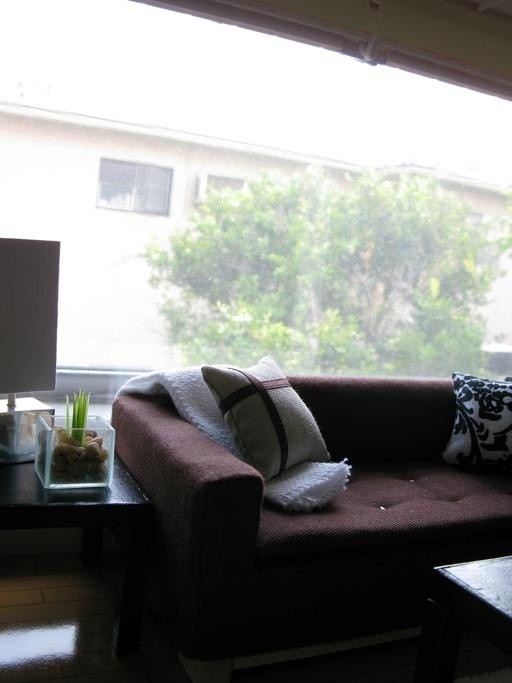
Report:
[111, 374, 512, 679]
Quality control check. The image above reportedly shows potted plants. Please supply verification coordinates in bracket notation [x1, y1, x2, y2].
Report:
[34, 387, 115, 490]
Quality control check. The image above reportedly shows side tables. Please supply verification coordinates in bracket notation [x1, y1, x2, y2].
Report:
[0, 450, 154, 659]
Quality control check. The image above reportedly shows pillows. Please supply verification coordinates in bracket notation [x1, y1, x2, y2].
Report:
[200, 355, 331, 485]
[443, 373, 512, 477]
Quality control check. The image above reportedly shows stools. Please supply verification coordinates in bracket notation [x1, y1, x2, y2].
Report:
[419, 554, 512, 680]
